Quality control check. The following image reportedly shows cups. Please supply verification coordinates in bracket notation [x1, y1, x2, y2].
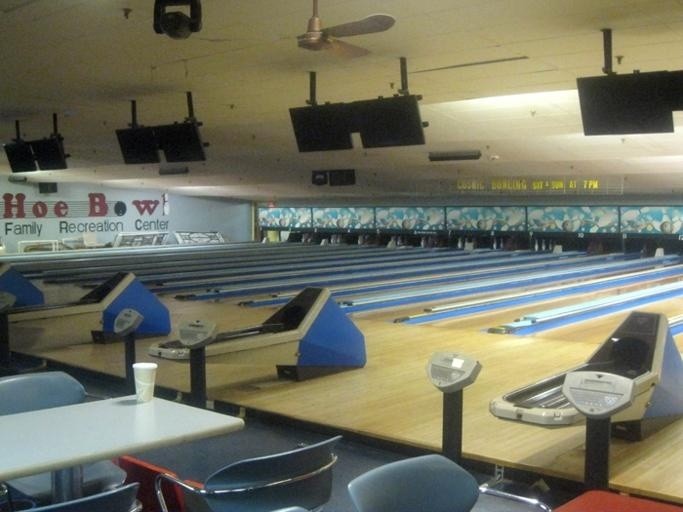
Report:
[130, 361, 158, 401]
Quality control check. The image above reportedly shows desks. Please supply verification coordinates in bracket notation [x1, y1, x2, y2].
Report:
[550, 488, 682, 512]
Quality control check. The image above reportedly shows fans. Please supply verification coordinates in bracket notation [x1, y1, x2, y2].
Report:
[296, 0, 395, 73]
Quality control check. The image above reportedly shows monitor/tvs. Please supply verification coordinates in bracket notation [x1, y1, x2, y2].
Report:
[156, 122, 207, 163]
[30, 138, 67, 170]
[3, 141, 37, 173]
[115, 126, 161, 165]
[350, 95, 426, 149]
[576, 70, 674, 136]
[289, 102, 354, 153]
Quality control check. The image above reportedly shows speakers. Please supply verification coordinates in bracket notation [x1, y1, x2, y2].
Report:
[329, 169, 356, 187]
[38, 182, 57, 194]
